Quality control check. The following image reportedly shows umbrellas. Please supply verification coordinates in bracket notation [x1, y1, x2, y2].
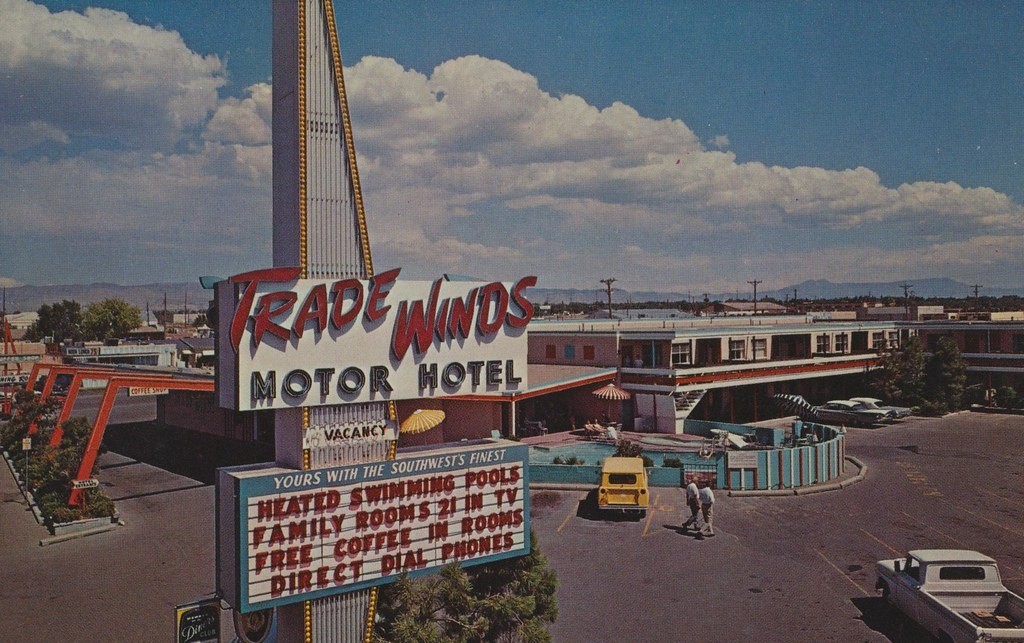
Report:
[400, 409, 445, 446]
[774, 393, 818, 422]
[591, 382, 631, 417]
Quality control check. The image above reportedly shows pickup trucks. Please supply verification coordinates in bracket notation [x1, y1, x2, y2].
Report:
[875, 550, 1024, 643]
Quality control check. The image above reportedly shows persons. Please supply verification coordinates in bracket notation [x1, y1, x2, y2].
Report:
[697, 479, 715, 540]
[623, 353, 644, 368]
[584, 418, 617, 440]
[681, 477, 701, 531]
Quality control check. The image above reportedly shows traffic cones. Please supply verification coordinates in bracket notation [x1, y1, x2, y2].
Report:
[812, 430, 818, 443]
[840, 423, 847, 433]
[723, 433, 730, 447]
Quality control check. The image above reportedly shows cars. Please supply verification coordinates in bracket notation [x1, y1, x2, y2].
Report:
[817, 397, 911, 427]
[597, 456, 651, 518]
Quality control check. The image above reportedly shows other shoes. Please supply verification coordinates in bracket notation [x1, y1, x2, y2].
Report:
[694, 526, 701, 530]
[710, 533, 715, 536]
[682, 523, 687, 530]
[696, 533, 703, 539]
